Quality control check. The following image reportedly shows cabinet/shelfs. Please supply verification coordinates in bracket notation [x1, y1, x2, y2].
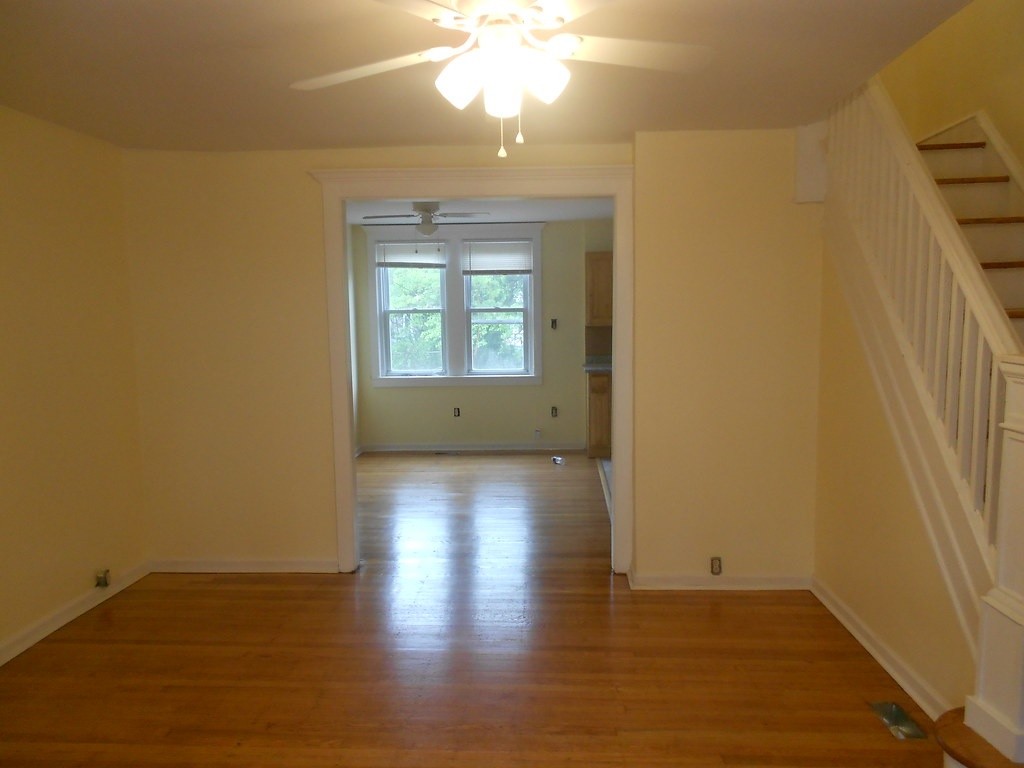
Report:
[587, 371, 612, 458]
[584, 251, 613, 327]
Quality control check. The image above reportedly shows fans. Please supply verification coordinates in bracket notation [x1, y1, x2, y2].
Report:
[287, 0, 712, 90]
[362, 201, 489, 220]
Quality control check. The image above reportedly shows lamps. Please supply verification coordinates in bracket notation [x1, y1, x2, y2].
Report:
[433, 24, 570, 160]
[415, 216, 441, 253]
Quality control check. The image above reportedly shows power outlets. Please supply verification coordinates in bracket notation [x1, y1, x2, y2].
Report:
[454, 408, 460, 417]
[551, 407, 557, 418]
[711, 557, 723, 575]
